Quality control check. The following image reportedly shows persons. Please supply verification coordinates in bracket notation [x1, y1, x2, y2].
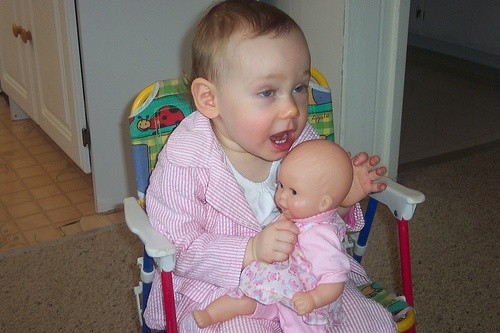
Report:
[193, 138, 355, 329]
[142, 0, 399, 333]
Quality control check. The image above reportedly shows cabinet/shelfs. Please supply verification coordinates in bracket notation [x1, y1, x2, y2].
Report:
[0, 0, 290, 214]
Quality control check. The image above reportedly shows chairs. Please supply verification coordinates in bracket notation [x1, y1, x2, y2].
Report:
[122, 67, 424, 333]
